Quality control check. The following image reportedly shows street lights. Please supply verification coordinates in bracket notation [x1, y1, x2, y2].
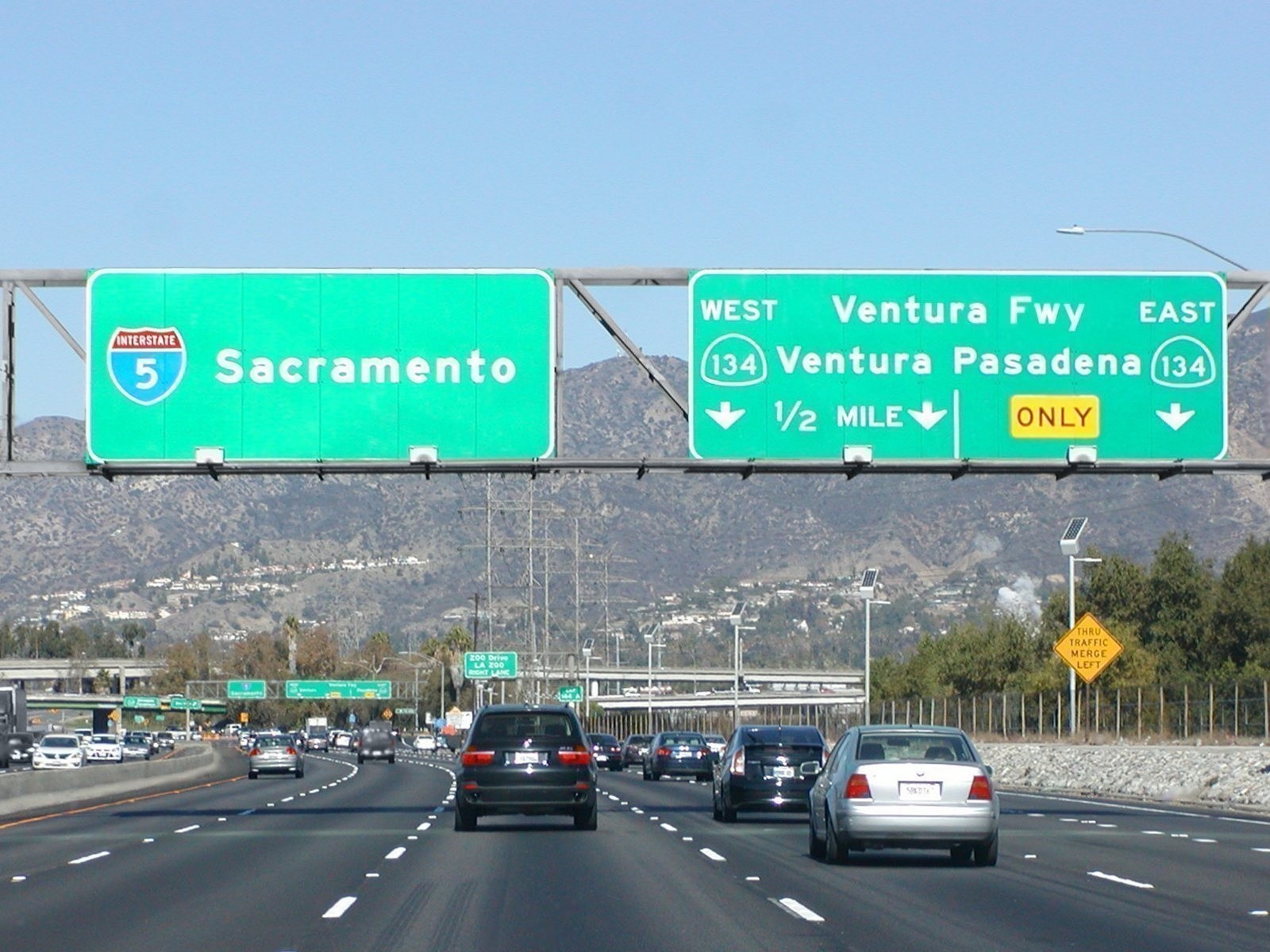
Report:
[581, 637, 603, 733]
[1058, 516, 1103, 738]
[858, 566, 893, 728]
[728, 599, 757, 733]
[643, 621, 667, 733]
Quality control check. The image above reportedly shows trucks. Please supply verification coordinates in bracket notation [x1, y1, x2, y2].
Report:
[0, 683, 28, 734]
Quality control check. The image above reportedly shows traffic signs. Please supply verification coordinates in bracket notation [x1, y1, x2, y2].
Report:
[686, 266, 1230, 470]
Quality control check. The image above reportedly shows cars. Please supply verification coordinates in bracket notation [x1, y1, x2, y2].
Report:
[72, 725, 203, 764]
[639, 731, 716, 783]
[30, 733, 90, 771]
[702, 724, 834, 824]
[618, 734, 653, 769]
[412, 734, 438, 754]
[217, 715, 402, 752]
[583, 733, 625, 772]
[702, 734, 728, 762]
[4, 732, 35, 767]
[800, 723, 1001, 870]
[247, 735, 307, 781]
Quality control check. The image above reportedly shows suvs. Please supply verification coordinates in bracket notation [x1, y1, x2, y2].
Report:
[354, 730, 397, 765]
[445, 700, 604, 831]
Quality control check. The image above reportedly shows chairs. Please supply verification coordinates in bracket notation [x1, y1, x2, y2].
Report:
[260, 741, 268, 747]
[925, 747, 953, 759]
[280, 740, 289, 745]
[860, 743, 885, 759]
[666, 739, 674, 744]
[488, 724, 508, 738]
[545, 723, 567, 735]
[690, 739, 699, 745]
